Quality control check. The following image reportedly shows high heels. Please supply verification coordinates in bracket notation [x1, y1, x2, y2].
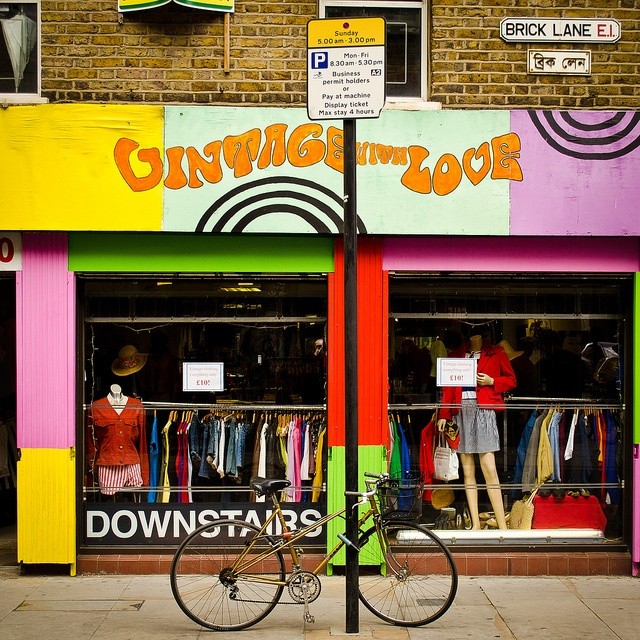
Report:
[478, 511, 507, 519]
[485, 513, 511, 527]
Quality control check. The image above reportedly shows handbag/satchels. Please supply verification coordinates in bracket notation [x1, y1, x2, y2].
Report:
[433, 429, 460, 480]
[511, 481, 541, 529]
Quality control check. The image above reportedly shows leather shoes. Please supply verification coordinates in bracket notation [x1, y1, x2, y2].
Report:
[551, 481, 565, 498]
[538, 479, 553, 497]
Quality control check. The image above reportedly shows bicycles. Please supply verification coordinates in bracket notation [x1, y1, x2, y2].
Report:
[170, 471, 459, 632]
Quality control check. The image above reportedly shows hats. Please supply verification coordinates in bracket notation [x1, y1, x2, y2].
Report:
[111, 345, 149, 376]
[498, 339, 523, 359]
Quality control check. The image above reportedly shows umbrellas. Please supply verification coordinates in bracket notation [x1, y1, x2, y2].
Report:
[1, 8, 35, 96]
